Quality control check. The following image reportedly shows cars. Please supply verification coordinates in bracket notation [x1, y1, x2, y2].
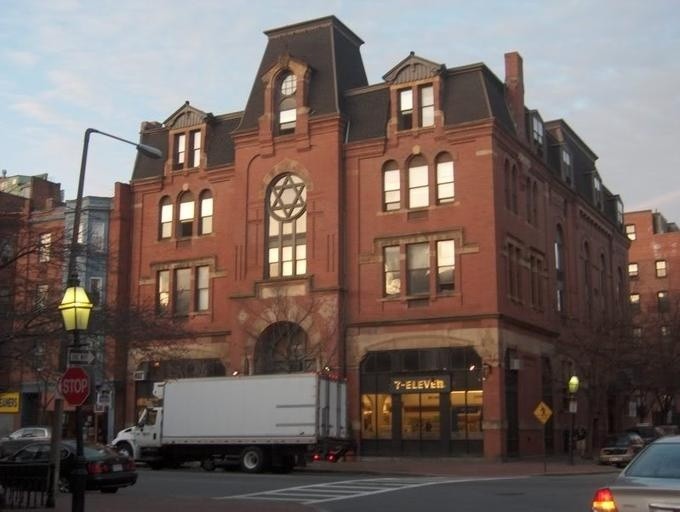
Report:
[0, 426, 51, 458]
[590, 424, 680, 512]
[0, 438, 138, 495]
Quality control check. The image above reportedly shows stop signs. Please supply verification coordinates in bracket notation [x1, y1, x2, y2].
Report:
[58, 367, 91, 408]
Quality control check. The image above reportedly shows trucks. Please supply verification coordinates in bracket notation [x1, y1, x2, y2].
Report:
[109, 373, 353, 473]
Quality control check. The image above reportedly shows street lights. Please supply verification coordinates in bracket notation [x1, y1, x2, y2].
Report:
[568, 376, 580, 465]
[44, 126, 163, 511]
[58, 273, 93, 511]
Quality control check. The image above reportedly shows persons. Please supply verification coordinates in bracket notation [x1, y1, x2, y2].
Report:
[575, 423, 587, 459]
